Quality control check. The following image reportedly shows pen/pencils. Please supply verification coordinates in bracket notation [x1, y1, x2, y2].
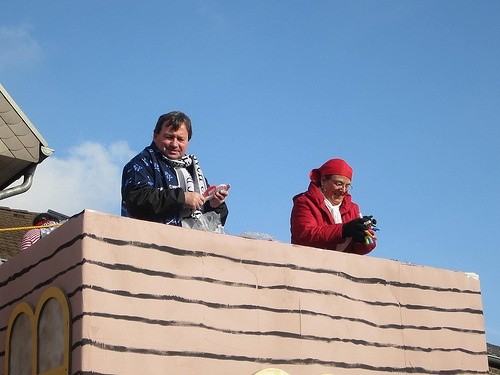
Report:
[363, 216, 380, 246]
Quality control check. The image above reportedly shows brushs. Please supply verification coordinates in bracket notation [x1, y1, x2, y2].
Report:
[203, 186, 216, 198]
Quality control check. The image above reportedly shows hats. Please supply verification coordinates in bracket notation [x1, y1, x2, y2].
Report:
[33, 213, 59, 226]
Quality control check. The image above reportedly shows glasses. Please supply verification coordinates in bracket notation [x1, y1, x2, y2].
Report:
[329, 177, 353, 190]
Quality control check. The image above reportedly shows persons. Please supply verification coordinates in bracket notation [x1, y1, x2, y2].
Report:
[290, 158, 377, 255]
[20, 213, 60, 252]
[121, 111, 231, 227]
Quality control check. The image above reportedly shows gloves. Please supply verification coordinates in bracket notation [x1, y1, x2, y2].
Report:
[342, 218, 371, 238]
[352, 215, 376, 242]
